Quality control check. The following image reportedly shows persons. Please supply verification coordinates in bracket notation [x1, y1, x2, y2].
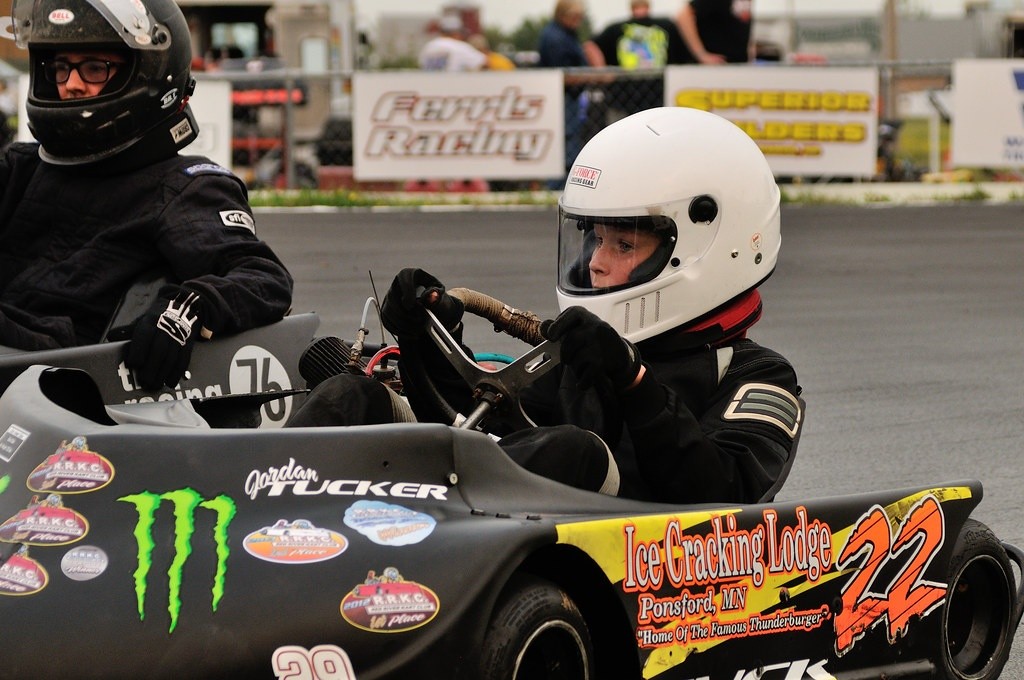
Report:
[468, 0, 752, 191]
[0, 0, 296, 395]
[287, 106, 805, 504]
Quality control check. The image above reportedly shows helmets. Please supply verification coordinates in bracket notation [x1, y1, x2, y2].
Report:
[26, 0, 194, 154]
[556, 105, 782, 344]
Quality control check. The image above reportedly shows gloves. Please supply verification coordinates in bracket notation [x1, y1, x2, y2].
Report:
[380, 267, 464, 340]
[123, 284, 204, 395]
[542, 306, 640, 386]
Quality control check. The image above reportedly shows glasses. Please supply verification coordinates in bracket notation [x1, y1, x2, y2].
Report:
[40, 57, 120, 85]
